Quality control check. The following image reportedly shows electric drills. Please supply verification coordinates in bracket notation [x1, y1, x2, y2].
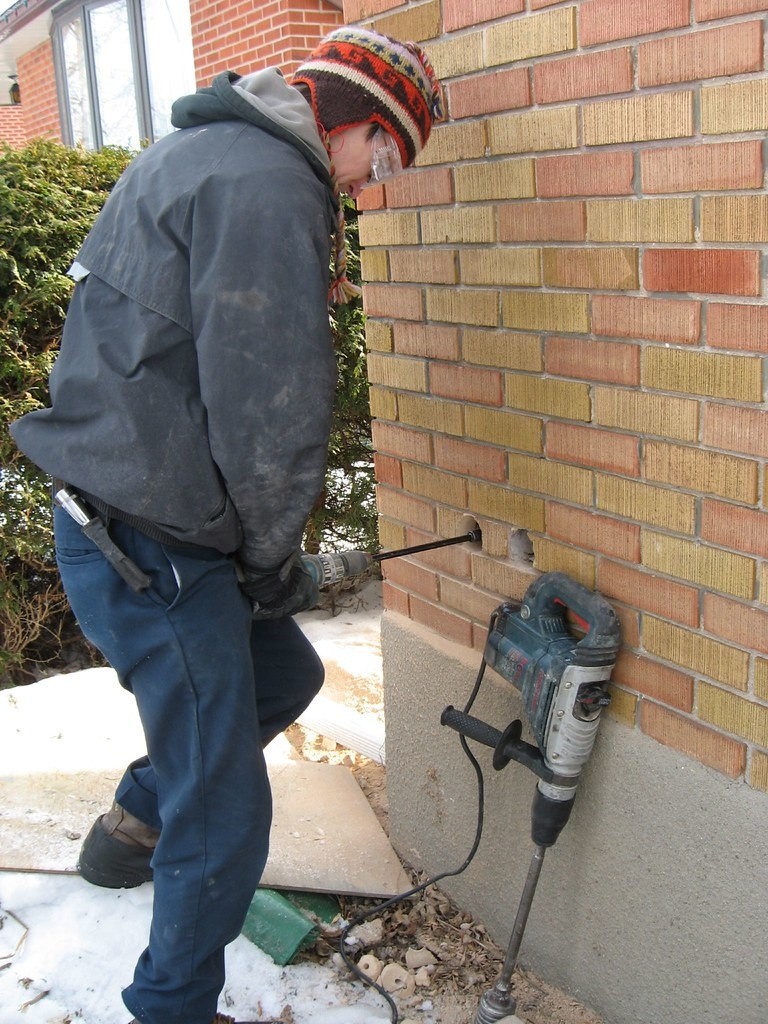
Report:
[439, 569, 623, 1024]
[302, 530, 481, 593]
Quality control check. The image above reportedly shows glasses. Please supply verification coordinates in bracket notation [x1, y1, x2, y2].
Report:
[359, 123, 402, 190]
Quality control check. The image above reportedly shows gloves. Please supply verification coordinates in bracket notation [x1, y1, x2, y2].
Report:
[239, 558, 321, 620]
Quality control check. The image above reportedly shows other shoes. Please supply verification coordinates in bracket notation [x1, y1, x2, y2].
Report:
[128, 1013, 285, 1024]
[77, 799, 162, 889]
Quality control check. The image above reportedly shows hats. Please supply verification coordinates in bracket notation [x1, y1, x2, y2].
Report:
[290, 28, 445, 304]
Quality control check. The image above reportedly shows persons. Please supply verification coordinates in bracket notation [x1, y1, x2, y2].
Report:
[8, 21, 441, 1024]
